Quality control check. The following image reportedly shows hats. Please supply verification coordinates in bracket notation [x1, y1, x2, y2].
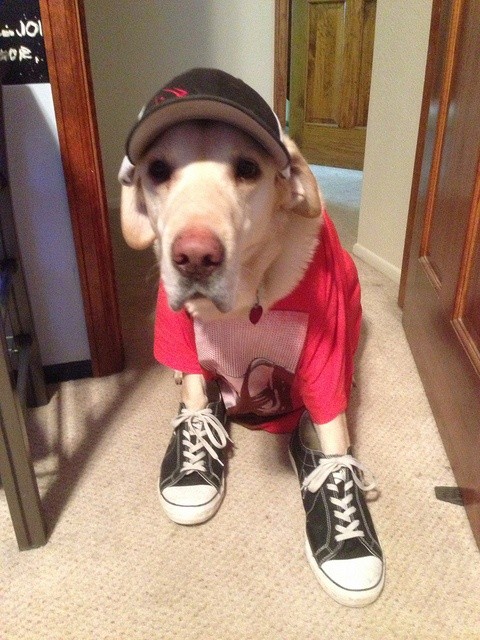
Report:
[125, 68, 291, 171]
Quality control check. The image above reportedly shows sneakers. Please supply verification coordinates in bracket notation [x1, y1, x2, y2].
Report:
[285, 410, 386, 609]
[156, 379, 231, 526]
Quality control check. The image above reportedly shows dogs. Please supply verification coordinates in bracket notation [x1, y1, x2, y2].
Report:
[117, 69, 362, 455]
